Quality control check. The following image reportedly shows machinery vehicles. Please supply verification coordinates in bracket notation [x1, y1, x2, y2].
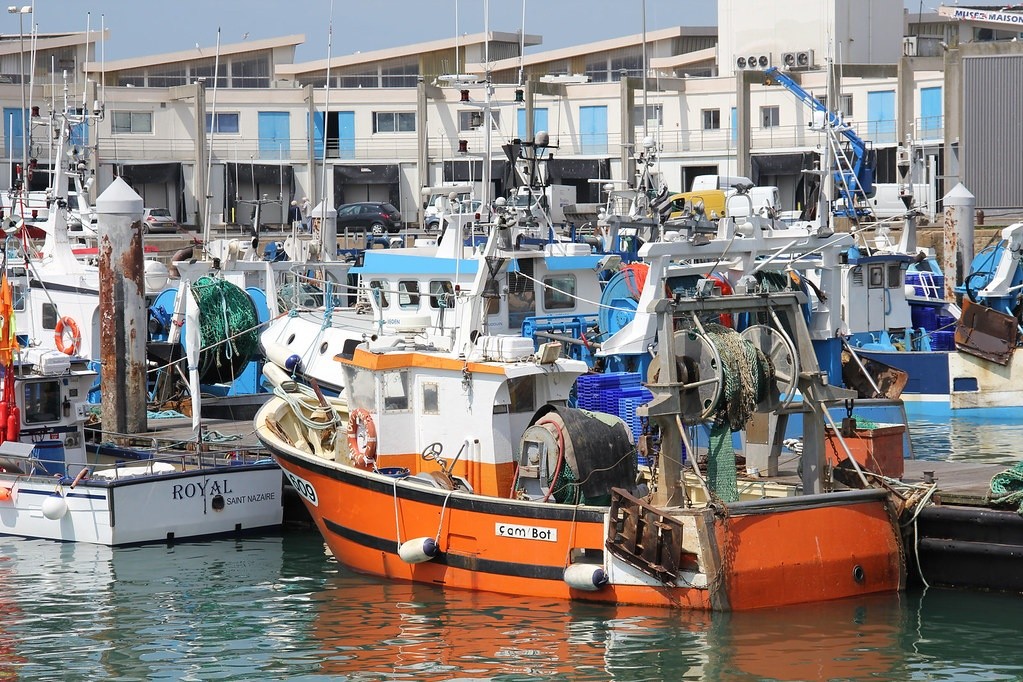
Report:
[763, 68, 876, 225]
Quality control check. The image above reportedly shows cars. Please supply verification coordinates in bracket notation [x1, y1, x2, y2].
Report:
[336, 201, 403, 236]
[141, 207, 178, 234]
[48, 192, 83, 232]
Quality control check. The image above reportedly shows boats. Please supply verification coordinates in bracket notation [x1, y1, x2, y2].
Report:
[0, 1, 1023, 609]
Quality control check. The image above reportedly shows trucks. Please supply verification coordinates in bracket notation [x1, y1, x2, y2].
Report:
[505, 183, 607, 228]
[422, 191, 495, 235]
[834, 184, 930, 230]
[648, 174, 782, 221]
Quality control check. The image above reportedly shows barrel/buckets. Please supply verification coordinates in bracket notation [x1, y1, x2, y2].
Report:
[32, 441, 65, 478]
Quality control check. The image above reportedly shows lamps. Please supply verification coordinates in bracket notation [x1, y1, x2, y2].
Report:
[899, 261, 909, 270]
[198, 77, 206, 82]
[693, 278, 716, 300]
[360, 167, 371, 172]
[692, 235, 712, 246]
[593, 255, 622, 274]
[665, 230, 681, 242]
[417, 76, 425, 83]
[619, 68, 629, 75]
[817, 226, 835, 239]
[939, 41, 949, 52]
[854, 264, 862, 274]
[645, 299, 671, 315]
[535, 341, 561, 364]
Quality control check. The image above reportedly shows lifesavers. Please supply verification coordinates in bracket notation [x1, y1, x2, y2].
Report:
[346, 408, 376, 466]
[55, 316, 80, 354]
[170, 248, 193, 279]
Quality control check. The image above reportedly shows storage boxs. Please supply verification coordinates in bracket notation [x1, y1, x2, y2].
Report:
[576, 371, 686, 465]
[905, 274, 944, 298]
[824, 421, 906, 483]
[913, 306, 955, 348]
